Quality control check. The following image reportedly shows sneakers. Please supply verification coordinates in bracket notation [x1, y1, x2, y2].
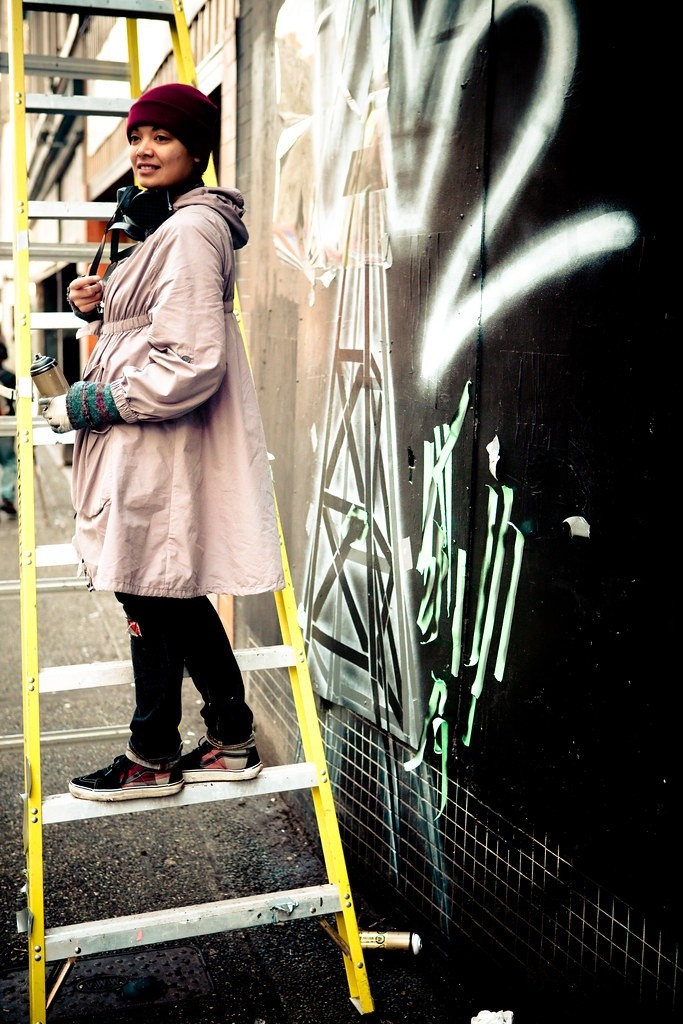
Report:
[180, 736, 263, 782]
[69, 754, 186, 801]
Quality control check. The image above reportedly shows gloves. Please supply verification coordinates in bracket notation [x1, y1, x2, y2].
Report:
[38, 394, 74, 433]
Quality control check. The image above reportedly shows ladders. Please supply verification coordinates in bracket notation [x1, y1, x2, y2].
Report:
[0, 0, 375, 1023]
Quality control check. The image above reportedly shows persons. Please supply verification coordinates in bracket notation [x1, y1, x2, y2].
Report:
[1, 334, 20, 516]
[37, 84, 285, 803]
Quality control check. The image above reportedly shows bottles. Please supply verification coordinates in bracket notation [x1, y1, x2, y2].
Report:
[30, 353, 70, 403]
[358, 930, 422, 955]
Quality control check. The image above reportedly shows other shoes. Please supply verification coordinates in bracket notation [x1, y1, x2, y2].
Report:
[0, 497, 18, 518]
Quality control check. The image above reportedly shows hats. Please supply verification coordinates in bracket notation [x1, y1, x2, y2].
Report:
[126, 83, 220, 176]
